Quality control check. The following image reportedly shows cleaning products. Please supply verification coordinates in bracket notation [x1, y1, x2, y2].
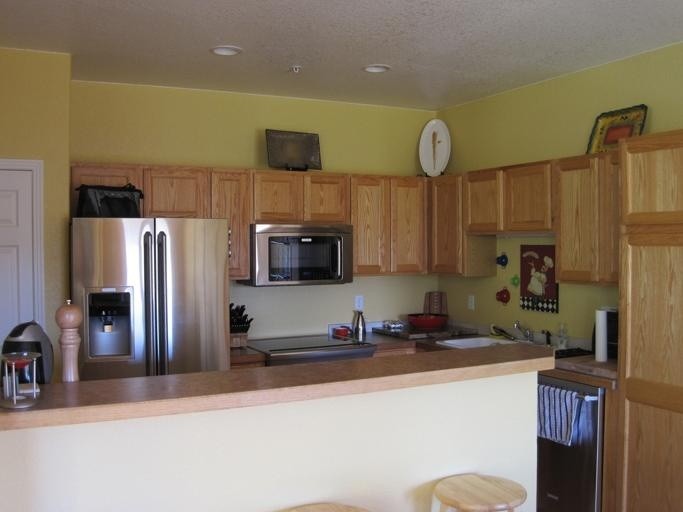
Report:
[555, 322, 568, 351]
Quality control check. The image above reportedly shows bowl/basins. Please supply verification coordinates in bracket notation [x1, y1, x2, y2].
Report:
[407, 313, 449, 331]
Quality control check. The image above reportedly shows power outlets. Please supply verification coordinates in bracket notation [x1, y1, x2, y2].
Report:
[467, 295, 475, 311]
[354, 295, 364, 310]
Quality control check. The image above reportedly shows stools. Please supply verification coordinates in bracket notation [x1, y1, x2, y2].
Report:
[434, 474, 527, 512]
[290, 502, 371, 512]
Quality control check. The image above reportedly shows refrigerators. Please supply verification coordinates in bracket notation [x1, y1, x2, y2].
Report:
[70, 216, 229, 379]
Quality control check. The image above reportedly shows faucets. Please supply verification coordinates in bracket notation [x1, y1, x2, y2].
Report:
[510, 318, 533, 340]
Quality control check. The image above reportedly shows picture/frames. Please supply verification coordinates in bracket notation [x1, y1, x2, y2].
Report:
[265, 128, 322, 170]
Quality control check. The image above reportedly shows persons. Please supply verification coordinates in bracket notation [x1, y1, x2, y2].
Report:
[526, 256, 553, 302]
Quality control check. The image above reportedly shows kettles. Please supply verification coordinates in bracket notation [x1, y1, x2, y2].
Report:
[1, 320, 54, 385]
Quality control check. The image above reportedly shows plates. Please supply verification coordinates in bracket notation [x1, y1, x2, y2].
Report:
[418, 118, 451, 179]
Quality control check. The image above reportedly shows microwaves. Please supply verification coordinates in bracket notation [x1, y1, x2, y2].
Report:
[250, 222, 354, 289]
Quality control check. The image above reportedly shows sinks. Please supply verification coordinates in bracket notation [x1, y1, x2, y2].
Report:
[436, 335, 518, 350]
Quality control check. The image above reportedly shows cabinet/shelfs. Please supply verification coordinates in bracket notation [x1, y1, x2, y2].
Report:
[143, 163, 211, 219]
[613, 128, 682, 512]
[390, 177, 430, 275]
[347, 333, 416, 357]
[351, 174, 391, 276]
[552, 149, 619, 288]
[504, 161, 553, 231]
[303, 172, 351, 223]
[71, 165, 143, 218]
[254, 170, 304, 224]
[211, 166, 252, 280]
[231, 347, 268, 369]
[430, 173, 497, 277]
[461, 168, 503, 235]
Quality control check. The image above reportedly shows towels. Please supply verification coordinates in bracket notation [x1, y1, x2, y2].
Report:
[533, 383, 580, 448]
